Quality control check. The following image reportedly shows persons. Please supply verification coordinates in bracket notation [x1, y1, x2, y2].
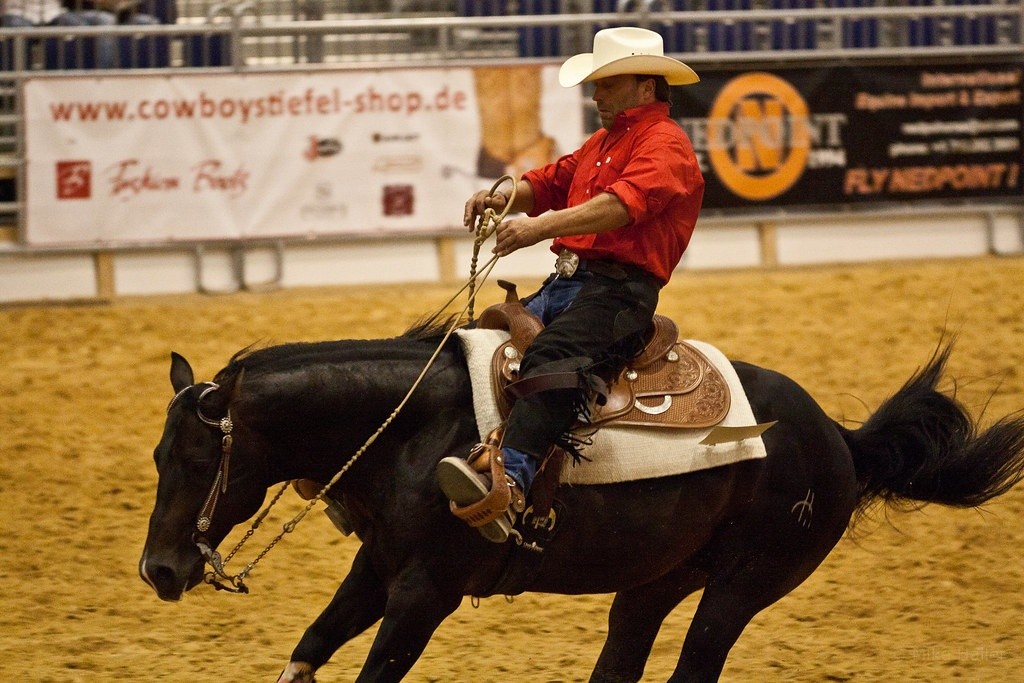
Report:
[439, 25, 706, 543]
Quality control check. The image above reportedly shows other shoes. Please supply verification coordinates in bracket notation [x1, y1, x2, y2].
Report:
[438, 458, 524, 543]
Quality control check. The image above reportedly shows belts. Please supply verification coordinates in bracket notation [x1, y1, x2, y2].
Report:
[554, 249, 660, 291]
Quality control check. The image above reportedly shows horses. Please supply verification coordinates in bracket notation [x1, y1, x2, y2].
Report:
[137, 309, 1023, 683]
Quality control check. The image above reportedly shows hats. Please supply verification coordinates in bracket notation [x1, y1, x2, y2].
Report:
[558, 26, 701, 87]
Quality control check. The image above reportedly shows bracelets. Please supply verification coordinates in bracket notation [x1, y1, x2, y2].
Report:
[496, 190, 509, 205]
